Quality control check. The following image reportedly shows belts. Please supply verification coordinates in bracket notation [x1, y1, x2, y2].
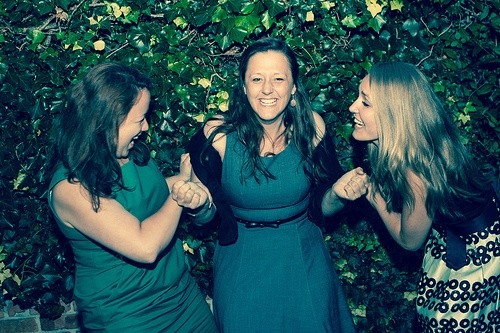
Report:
[235, 207, 307, 229]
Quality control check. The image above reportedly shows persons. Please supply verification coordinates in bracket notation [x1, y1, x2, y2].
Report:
[348, 63, 500, 333]
[39, 60, 224, 332]
[172, 39, 370, 332]
